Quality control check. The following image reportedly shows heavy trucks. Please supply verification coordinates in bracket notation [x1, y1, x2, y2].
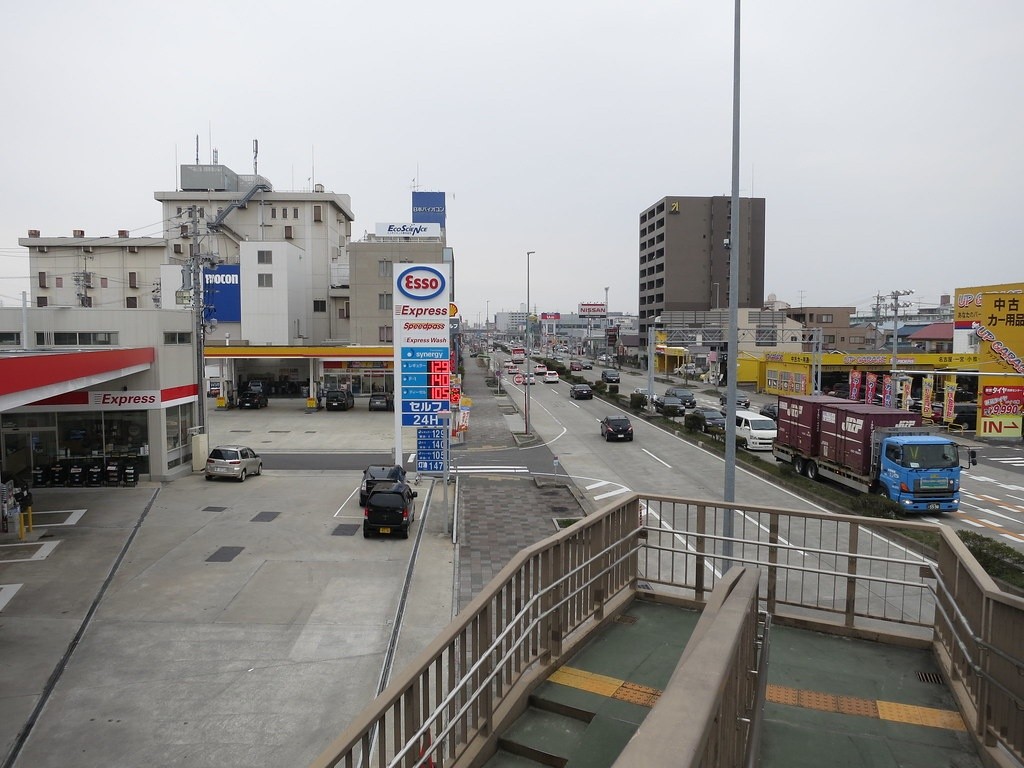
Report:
[773, 394, 977, 513]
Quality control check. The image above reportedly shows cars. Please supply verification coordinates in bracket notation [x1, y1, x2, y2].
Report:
[720, 389, 750, 408]
[570, 384, 593, 399]
[600, 415, 633, 441]
[239, 391, 268, 409]
[673, 364, 695, 374]
[761, 402, 779, 420]
[369, 393, 394, 410]
[634, 386, 725, 433]
[359, 464, 406, 506]
[504, 347, 594, 385]
[466, 335, 502, 353]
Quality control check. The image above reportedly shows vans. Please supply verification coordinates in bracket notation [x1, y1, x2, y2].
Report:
[602, 369, 620, 383]
[735, 410, 778, 451]
[364, 482, 417, 539]
[326, 390, 354, 411]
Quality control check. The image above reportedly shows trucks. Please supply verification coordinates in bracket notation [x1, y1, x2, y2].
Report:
[512, 348, 524, 364]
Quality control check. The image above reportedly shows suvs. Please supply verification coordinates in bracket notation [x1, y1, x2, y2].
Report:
[205, 445, 262, 481]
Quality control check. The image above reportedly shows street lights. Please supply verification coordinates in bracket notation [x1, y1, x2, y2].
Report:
[486, 301, 490, 354]
[527, 251, 539, 434]
